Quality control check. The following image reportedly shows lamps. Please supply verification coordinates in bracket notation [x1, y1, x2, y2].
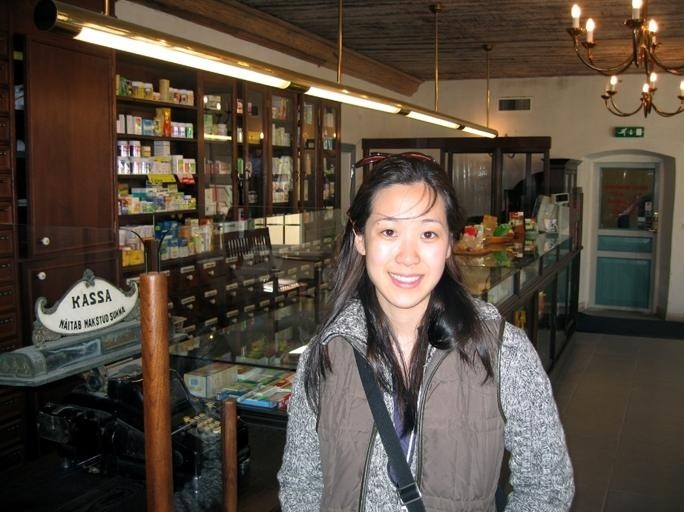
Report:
[566, 0, 684, 121]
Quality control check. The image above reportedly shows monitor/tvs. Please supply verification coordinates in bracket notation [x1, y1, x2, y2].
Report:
[530, 194, 555, 231]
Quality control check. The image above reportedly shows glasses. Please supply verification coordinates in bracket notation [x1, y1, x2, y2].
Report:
[349, 151, 435, 216]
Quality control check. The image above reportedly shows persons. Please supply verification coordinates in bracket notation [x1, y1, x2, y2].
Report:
[279, 153, 575, 512]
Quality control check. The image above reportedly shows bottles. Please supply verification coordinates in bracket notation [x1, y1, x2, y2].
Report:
[171, 122, 193, 139]
[132, 79, 195, 107]
[121, 241, 144, 266]
[116, 140, 151, 175]
[171, 155, 196, 175]
[323, 163, 335, 200]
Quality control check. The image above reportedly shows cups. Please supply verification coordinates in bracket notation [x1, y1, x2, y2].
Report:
[543, 218, 557, 234]
